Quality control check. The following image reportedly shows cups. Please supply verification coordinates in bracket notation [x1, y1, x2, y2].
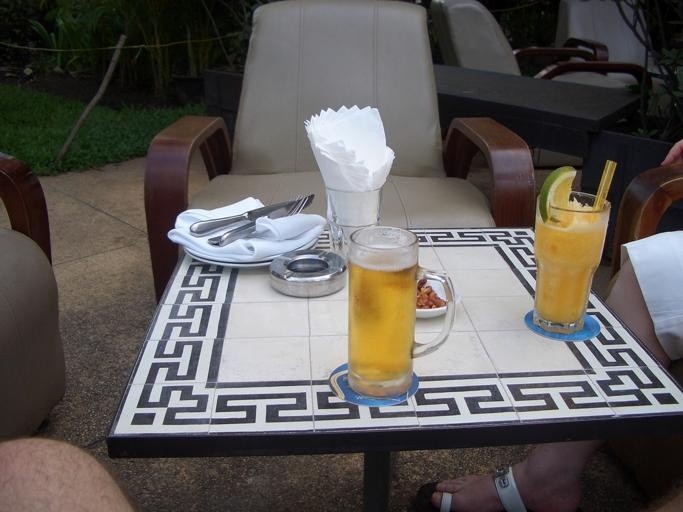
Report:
[324, 186, 381, 263]
[533, 190, 611, 334]
[345, 227, 454, 397]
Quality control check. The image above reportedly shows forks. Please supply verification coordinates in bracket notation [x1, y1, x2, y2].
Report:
[209, 195, 307, 245]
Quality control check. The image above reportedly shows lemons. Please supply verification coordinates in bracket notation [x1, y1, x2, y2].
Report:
[538, 166, 577, 223]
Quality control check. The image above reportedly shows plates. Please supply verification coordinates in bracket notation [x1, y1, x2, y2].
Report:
[416, 280, 463, 321]
[183, 236, 318, 268]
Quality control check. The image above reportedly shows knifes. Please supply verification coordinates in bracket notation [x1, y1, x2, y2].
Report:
[188, 193, 315, 238]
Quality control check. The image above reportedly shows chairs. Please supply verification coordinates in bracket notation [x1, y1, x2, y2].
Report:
[606, 159, 682, 279]
[549, 1, 672, 90]
[426, 0, 654, 102]
[0, 151, 78, 444]
[138, 1, 540, 314]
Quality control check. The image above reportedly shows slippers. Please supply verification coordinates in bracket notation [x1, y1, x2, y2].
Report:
[417, 465, 533, 511]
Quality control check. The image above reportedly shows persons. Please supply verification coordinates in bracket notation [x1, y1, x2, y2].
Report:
[0, 438, 134, 512]
[415, 139, 683, 512]
